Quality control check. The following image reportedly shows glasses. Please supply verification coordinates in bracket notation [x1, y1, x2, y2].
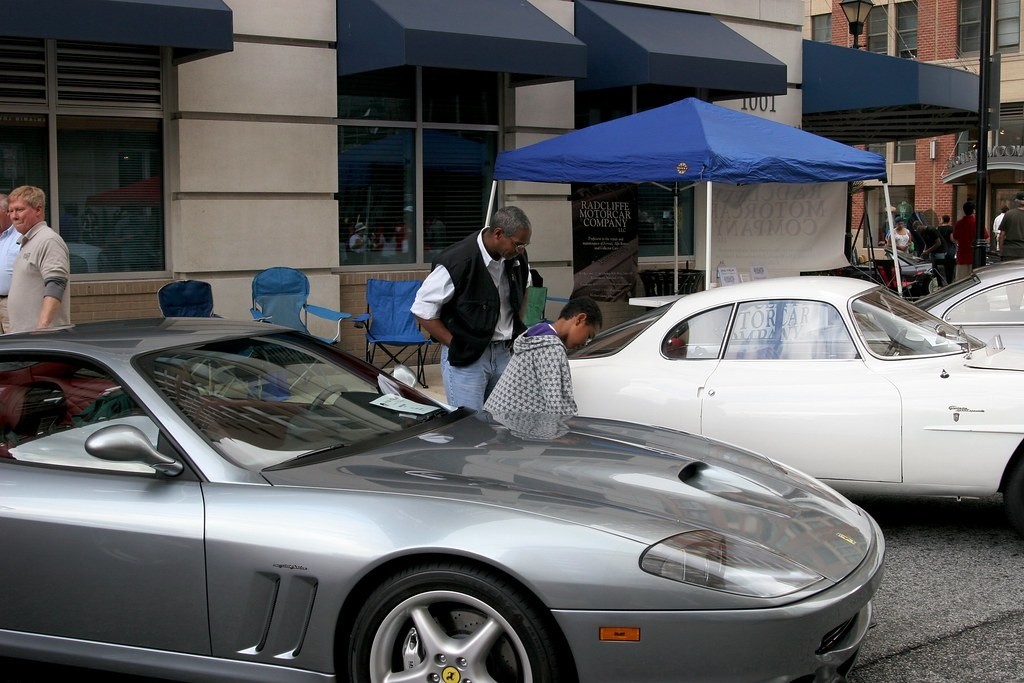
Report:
[506, 232, 530, 252]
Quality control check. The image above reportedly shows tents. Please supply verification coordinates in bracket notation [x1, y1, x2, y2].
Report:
[483, 98, 903, 297]
[338, 129, 489, 265]
[82, 176, 161, 228]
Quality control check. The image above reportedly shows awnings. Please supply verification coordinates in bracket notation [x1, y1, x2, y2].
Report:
[337, 0, 587, 88]
[0, 0, 233, 66]
[574, 0, 787, 101]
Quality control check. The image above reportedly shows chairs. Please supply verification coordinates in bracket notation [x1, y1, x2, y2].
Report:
[3, 380, 72, 442]
[872, 259, 924, 302]
[156, 279, 223, 319]
[249, 265, 352, 401]
[353, 277, 434, 389]
[521, 286, 549, 328]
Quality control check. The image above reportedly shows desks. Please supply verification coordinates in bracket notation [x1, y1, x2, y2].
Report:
[628, 294, 686, 308]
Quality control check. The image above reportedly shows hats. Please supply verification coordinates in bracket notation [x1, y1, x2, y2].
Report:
[1016, 193, 1024, 200]
[895, 216, 905, 222]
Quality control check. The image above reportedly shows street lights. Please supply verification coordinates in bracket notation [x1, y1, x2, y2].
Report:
[839, 0, 877, 263]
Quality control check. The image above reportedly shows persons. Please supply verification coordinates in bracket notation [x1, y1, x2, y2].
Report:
[8, 185, 71, 368]
[410, 206, 533, 414]
[885, 192, 1024, 311]
[481, 296, 603, 417]
[417, 415, 592, 451]
[62, 203, 160, 273]
[339, 207, 447, 265]
[0, 193, 22, 370]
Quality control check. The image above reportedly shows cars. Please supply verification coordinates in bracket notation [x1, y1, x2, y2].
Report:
[565, 274, 1024, 535]
[809, 257, 1024, 349]
[0, 317, 888, 682]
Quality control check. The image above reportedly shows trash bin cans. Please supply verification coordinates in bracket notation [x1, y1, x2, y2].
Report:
[638, 268, 706, 312]
